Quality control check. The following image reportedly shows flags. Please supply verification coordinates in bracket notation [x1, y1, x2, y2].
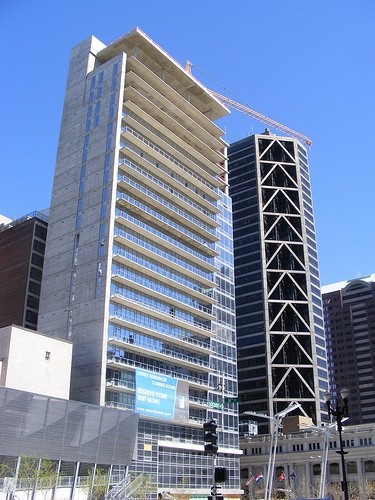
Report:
[279, 470, 297, 482]
[245, 470, 263, 486]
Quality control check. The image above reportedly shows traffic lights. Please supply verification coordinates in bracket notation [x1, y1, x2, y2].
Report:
[202, 419, 219, 456]
[214, 466, 227, 482]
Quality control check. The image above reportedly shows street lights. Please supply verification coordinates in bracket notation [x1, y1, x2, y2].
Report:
[322, 386, 350, 500]
